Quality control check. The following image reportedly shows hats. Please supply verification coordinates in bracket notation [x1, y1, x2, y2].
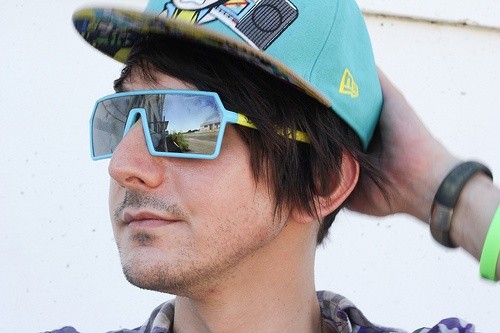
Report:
[72, 1, 383, 151]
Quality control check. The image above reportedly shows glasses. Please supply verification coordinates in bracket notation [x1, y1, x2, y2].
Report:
[89, 90, 309, 160]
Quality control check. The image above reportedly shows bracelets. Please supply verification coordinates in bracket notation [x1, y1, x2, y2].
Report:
[429, 160, 493, 249]
[479, 204, 500, 282]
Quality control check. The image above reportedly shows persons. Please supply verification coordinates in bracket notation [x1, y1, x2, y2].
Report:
[42, 0, 500, 332]
